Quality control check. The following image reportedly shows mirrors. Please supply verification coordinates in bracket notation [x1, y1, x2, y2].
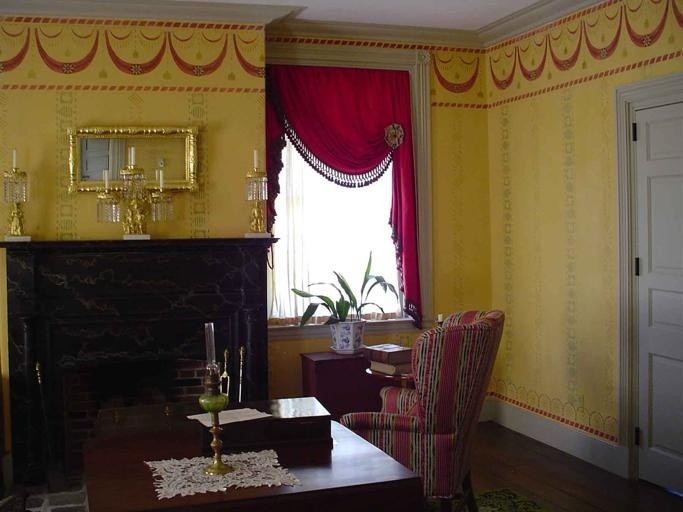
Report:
[67, 126, 200, 195]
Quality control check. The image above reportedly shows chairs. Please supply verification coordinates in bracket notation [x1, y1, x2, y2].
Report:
[339, 310, 505, 512]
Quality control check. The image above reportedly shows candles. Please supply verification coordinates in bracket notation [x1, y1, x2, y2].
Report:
[156, 170, 158, 180]
[131, 145, 135, 165]
[105, 170, 108, 189]
[254, 149, 258, 168]
[160, 170, 164, 187]
[12, 148, 17, 169]
[103, 169, 105, 180]
[128, 146, 131, 165]
[438, 314, 443, 322]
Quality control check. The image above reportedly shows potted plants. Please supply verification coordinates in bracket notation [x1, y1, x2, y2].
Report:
[292, 252, 398, 354]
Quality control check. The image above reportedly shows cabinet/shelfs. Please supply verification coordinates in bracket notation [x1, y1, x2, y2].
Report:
[299, 352, 382, 422]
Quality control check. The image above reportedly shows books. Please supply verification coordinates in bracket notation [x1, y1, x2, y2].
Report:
[370, 360, 412, 376]
[362, 343, 413, 365]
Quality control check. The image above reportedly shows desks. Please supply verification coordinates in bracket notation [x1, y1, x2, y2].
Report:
[366, 368, 415, 389]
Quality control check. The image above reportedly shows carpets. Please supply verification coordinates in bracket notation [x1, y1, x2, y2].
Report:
[423, 488, 554, 512]
